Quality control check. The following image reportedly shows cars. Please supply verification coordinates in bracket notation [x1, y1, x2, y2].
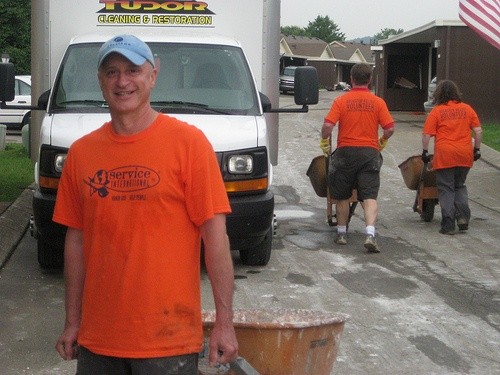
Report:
[0, 75, 45, 131]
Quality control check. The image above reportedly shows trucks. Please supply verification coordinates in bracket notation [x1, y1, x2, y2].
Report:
[0, 1, 318, 276]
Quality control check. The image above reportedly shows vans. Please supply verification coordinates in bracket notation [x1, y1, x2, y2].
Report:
[279, 66, 307, 94]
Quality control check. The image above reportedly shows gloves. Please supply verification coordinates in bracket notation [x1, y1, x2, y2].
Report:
[473, 147, 481, 161]
[319, 136, 331, 156]
[422, 149, 434, 163]
[378, 135, 387, 151]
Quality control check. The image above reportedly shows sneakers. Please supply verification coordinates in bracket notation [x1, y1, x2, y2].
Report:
[364, 235, 381, 252]
[336, 232, 348, 244]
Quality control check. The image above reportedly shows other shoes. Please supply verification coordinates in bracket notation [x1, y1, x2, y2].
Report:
[438, 218, 468, 235]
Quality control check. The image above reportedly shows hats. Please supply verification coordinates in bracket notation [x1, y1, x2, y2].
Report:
[96, 33, 155, 69]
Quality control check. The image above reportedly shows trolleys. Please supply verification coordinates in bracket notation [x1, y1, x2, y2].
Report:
[305, 131, 383, 228]
[398, 147, 481, 222]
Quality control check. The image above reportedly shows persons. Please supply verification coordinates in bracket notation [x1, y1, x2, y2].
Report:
[319, 63, 394, 253]
[52, 36, 238, 374]
[421, 80, 484, 237]
